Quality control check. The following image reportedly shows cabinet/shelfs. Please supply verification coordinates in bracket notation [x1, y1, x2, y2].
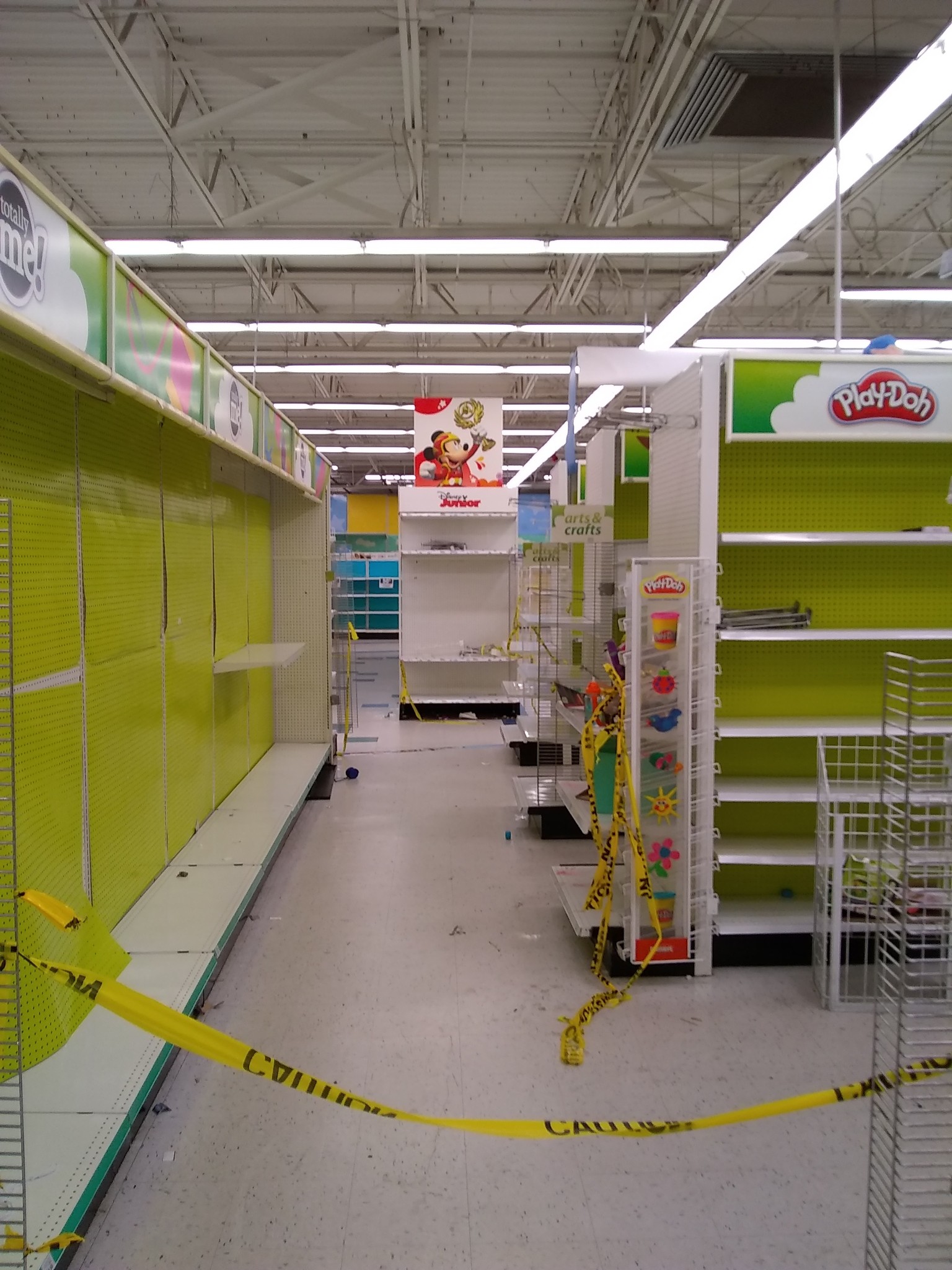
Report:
[333, 551, 398, 634]
[0, 148, 339, 1270]
[527, 346, 952, 977]
[398, 486, 522, 719]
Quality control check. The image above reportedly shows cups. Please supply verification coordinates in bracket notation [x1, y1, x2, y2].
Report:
[651, 612, 680, 651]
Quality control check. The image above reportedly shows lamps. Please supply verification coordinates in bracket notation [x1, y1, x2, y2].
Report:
[86, 0, 952, 485]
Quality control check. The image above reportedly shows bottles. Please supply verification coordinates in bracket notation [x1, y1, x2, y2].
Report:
[584, 676, 601, 723]
